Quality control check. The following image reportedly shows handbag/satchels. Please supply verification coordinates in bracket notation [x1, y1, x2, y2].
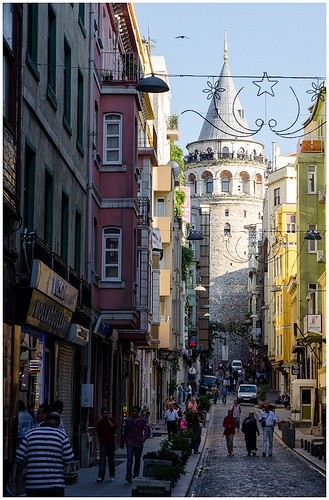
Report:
[261, 421, 266, 428]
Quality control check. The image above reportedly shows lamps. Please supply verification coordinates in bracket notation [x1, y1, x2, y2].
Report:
[136, 73, 171, 96]
[270, 286, 283, 293]
[203, 304, 211, 308]
[260, 305, 270, 310]
[193, 284, 207, 292]
[245, 320, 251, 323]
[204, 313, 211, 317]
[303, 227, 323, 230]
[186, 231, 204, 241]
[305, 289, 315, 302]
[250, 314, 258, 318]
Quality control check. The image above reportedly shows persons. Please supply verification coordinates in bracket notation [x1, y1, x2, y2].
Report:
[121, 406, 149, 482]
[141, 364, 290, 458]
[15, 399, 75, 497]
[97, 409, 119, 483]
[17, 401, 35, 442]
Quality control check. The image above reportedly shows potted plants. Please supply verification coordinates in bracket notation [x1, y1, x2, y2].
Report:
[131, 428, 195, 496]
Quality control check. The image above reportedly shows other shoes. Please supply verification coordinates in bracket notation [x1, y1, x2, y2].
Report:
[96, 477, 115, 482]
[227, 452, 234, 457]
[247, 450, 257, 456]
[262, 452, 272, 457]
[125, 473, 139, 483]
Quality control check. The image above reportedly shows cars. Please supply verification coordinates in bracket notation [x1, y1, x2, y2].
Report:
[237, 383, 258, 406]
[232, 360, 244, 371]
[199, 374, 219, 393]
